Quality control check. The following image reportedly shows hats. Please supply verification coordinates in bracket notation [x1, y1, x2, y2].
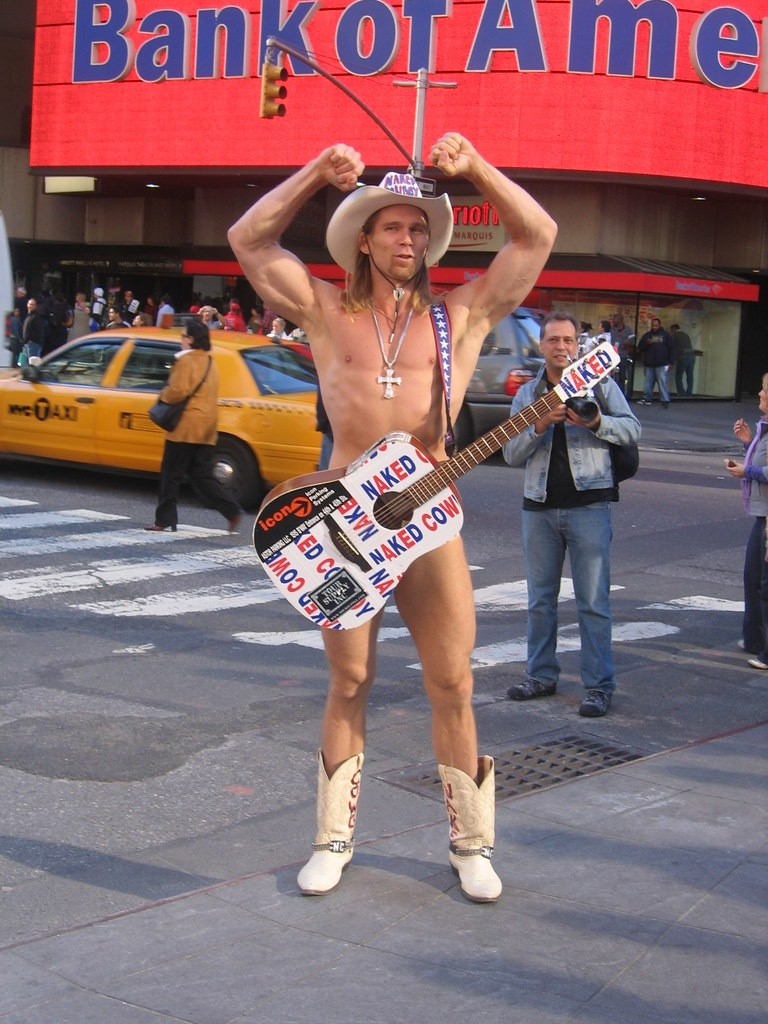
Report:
[94, 288, 103, 296]
[230, 304, 240, 310]
[326, 172, 453, 276]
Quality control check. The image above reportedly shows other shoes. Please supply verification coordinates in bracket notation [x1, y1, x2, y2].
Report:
[737, 640, 745, 648]
[747, 658, 768, 669]
[636, 399, 651, 405]
[507, 679, 556, 699]
[579, 690, 611, 717]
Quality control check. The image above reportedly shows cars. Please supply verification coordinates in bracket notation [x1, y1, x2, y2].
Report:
[452, 307, 546, 454]
[0, 326, 323, 511]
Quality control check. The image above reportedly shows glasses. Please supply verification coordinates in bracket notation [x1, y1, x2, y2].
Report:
[181, 333, 190, 338]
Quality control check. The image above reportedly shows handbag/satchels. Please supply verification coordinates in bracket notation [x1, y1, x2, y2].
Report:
[593, 383, 638, 482]
[147, 399, 184, 430]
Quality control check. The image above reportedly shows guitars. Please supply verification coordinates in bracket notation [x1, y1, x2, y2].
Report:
[251, 340, 622, 632]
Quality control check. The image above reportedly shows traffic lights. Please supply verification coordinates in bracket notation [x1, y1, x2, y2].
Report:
[259, 63, 287, 119]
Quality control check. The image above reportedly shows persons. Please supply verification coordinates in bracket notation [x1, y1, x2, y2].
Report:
[579, 315, 694, 409]
[310, 391, 333, 472]
[7, 286, 308, 368]
[724, 373, 768, 669]
[145, 318, 245, 533]
[506, 313, 641, 718]
[226, 132, 557, 906]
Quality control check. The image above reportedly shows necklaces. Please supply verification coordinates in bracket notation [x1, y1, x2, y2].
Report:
[367, 306, 415, 401]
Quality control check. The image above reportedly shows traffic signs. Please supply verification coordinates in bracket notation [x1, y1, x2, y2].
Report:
[414, 175, 436, 198]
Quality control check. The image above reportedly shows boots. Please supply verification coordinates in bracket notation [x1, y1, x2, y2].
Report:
[297, 752, 364, 895]
[438, 754, 503, 902]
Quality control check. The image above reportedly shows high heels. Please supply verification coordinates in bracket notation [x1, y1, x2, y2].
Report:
[229, 510, 241, 532]
[144, 523, 176, 531]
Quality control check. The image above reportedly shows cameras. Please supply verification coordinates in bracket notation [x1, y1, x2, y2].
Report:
[565, 398, 599, 423]
